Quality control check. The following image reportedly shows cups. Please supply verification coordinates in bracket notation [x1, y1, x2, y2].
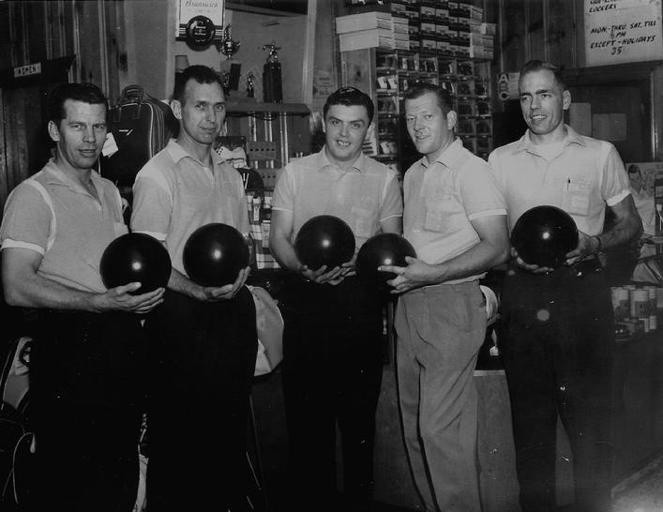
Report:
[374, 54, 489, 176]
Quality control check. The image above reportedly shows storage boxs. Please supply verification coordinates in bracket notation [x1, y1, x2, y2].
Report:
[332, 1, 497, 59]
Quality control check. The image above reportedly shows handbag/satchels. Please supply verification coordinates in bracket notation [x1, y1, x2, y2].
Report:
[97, 84, 180, 198]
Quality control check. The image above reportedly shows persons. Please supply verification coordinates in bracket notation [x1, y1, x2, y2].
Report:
[269, 88, 404, 511]
[376, 83, 512, 510]
[488, 60, 644, 512]
[130, 64, 258, 511]
[628, 164, 656, 237]
[0, 82, 165, 512]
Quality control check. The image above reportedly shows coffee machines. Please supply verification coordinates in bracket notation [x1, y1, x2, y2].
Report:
[219, 102, 314, 188]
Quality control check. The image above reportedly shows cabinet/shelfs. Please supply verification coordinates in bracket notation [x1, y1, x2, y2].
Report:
[609, 252, 663, 502]
[337, 51, 494, 180]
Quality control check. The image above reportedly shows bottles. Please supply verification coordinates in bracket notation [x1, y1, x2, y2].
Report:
[259, 41, 284, 104]
[245, 190, 272, 263]
[611, 283, 662, 334]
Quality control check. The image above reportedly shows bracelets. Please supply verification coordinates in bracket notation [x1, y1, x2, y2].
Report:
[590, 236, 602, 253]
